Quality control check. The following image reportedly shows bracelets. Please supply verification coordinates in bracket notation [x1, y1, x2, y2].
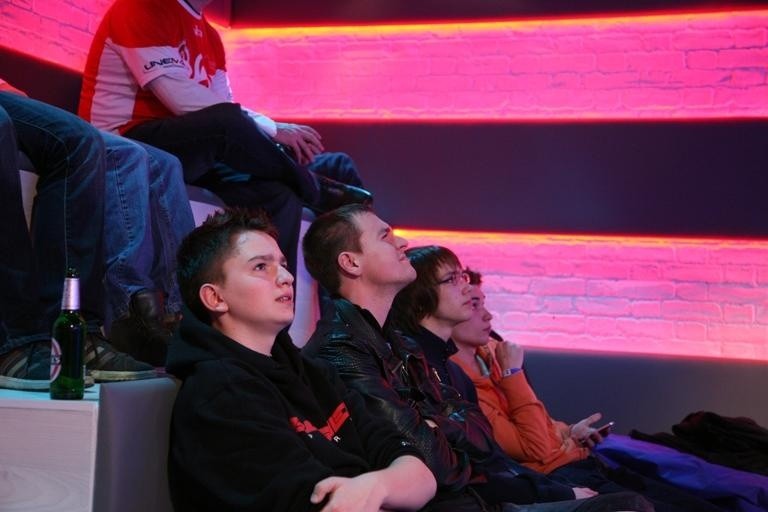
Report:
[500, 367, 521, 376]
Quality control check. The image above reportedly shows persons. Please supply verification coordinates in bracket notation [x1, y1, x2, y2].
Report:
[167, 208, 441, 510]
[297, 202, 654, 511]
[2, 90, 161, 392]
[76, 0, 375, 360]
[447, 265, 729, 510]
[390, 244, 601, 504]
[0, 77, 197, 366]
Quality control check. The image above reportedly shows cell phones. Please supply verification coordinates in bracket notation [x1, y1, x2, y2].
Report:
[578, 421, 617, 445]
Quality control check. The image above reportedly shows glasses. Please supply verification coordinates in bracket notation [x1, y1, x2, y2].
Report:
[434, 271, 471, 287]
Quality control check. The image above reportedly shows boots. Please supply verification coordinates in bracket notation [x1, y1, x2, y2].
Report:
[108, 284, 176, 365]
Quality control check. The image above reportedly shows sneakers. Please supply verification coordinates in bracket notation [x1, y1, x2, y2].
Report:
[82, 333, 158, 382]
[314, 170, 373, 210]
[0, 340, 94, 389]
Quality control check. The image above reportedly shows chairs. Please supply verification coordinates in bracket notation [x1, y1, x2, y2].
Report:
[0, 145, 319, 512]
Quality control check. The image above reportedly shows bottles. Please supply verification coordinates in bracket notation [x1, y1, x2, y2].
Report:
[50, 268, 88, 401]
